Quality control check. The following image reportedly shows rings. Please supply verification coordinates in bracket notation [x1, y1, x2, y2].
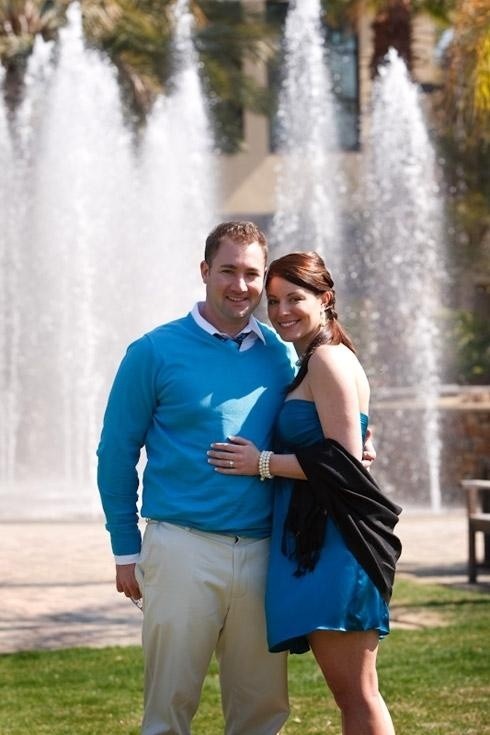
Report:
[229, 460, 234, 468]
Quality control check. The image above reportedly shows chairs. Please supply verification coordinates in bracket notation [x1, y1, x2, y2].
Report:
[459, 479, 490, 585]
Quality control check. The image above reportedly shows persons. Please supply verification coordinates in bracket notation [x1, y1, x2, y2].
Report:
[95, 220, 378, 735]
[203, 249, 401, 735]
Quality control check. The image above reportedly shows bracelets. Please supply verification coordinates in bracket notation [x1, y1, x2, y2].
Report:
[258, 450, 273, 482]
